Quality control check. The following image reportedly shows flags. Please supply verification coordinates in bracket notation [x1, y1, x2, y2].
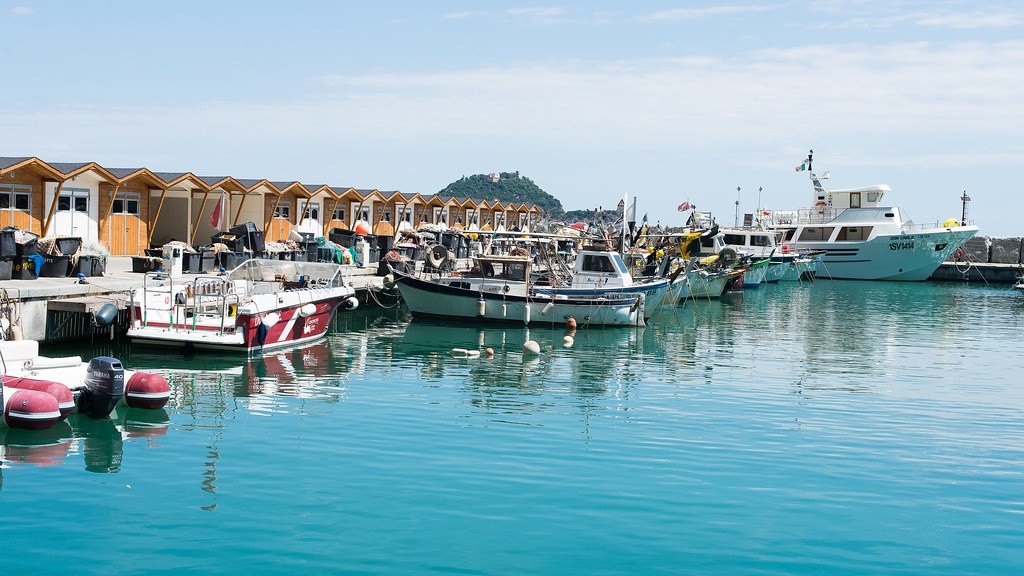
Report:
[793, 163, 809, 174]
[209, 191, 228, 232]
[677, 200, 690, 214]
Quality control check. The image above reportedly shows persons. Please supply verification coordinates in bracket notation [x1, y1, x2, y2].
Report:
[953, 244, 963, 262]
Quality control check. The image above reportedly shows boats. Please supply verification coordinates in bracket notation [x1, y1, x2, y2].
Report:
[126, 258, 360, 356]
[0, 324, 173, 429]
[110, 330, 368, 399]
[764, 148, 979, 282]
[389, 230, 827, 328]
